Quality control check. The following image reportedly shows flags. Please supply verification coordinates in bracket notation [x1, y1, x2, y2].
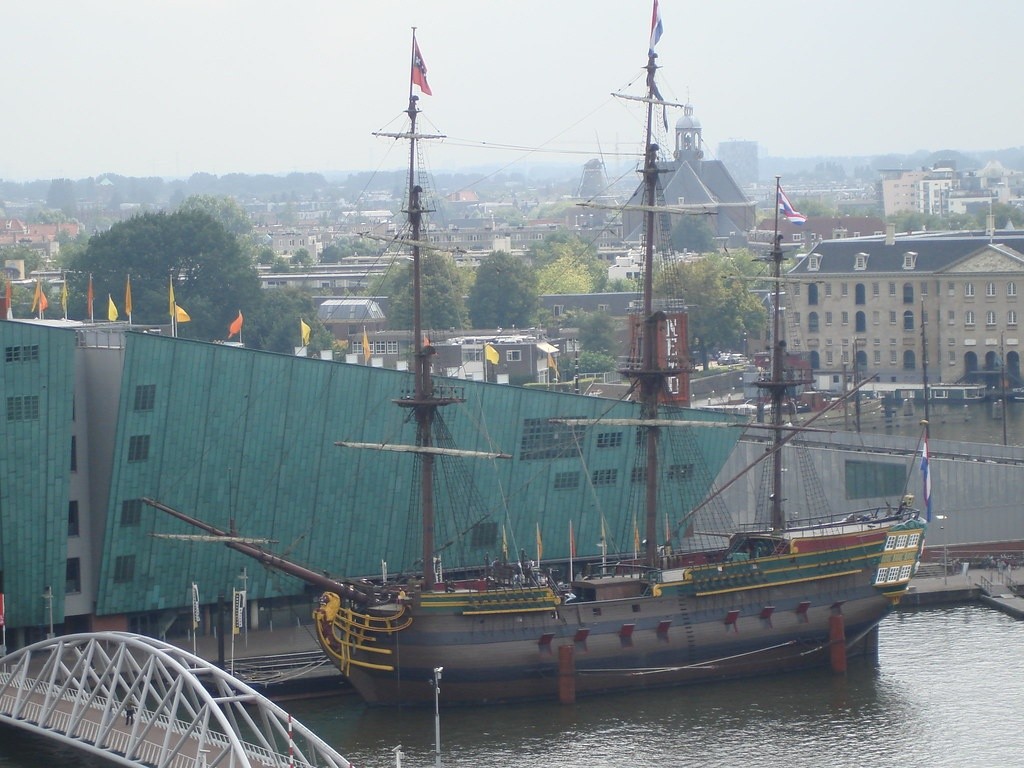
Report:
[502, 528, 509, 559]
[548, 354, 557, 371]
[5, 283, 12, 310]
[412, 38, 432, 96]
[40, 287, 47, 311]
[649, 6, 664, 50]
[634, 522, 640, 553]
[364, 329, 370, 364]
[169, 280, 191, 322]
[229, 311, 244, 337]
[301, 320, 310, 346]
[778, 187, 807, 226]
[920, 444, 932, 522]
[665, 520, 672, 554]
[536, 527, 543, 559]
[31, 282, 40, 312]
[108, 298, 119, 321]
[485, 344, 499, 366]
[570, 522, 576, 558]
[125, 277, 132, 315]
[88, 279, 94, 315]
[62, 282, 68, 307]
[424, 336, 429, 347]
[601, 520, 607, 550]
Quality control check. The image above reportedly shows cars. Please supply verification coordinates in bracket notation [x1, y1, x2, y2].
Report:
[732, 353, 747, 364]
[716, 355, 735, 365]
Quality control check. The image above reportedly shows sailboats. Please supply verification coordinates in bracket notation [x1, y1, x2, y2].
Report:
[140, 0, 930, 709]
